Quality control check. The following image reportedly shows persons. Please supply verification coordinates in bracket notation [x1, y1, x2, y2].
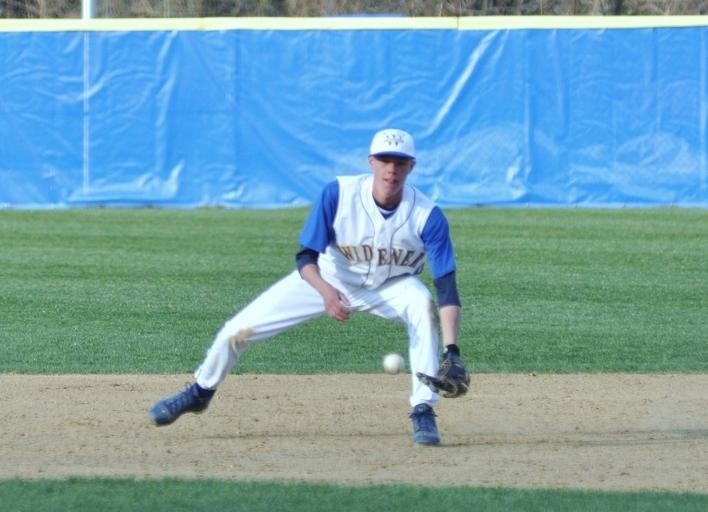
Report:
[148, 129, 470, 445]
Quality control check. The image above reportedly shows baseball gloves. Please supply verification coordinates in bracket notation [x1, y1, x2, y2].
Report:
[417, 343, 468, 397]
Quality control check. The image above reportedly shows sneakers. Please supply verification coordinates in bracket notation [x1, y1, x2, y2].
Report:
[409, 403, 444, 447]
[149, 381, 222, 428]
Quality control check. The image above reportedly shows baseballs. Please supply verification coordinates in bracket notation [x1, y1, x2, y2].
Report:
[383, 352, 403, 374]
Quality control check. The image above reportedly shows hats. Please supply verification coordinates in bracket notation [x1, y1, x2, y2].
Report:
[366, 127, 417, 162]
